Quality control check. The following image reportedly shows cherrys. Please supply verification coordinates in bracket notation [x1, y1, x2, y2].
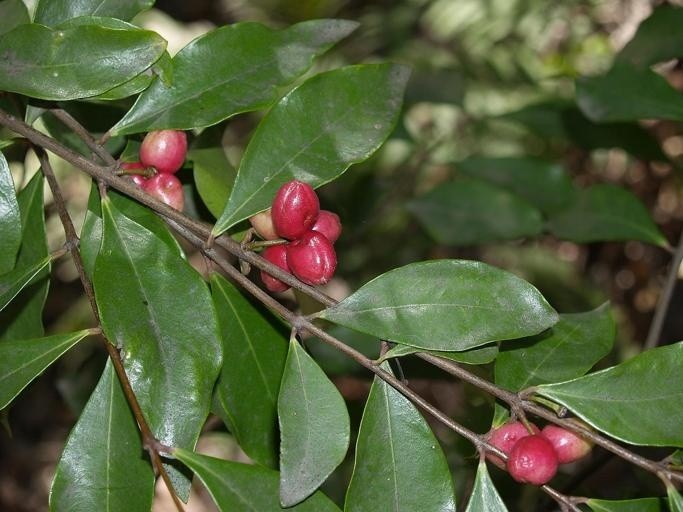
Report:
[486, 420, 595, 486]
[247, 181, 342, 292]
[120, 129, 188, 212]
